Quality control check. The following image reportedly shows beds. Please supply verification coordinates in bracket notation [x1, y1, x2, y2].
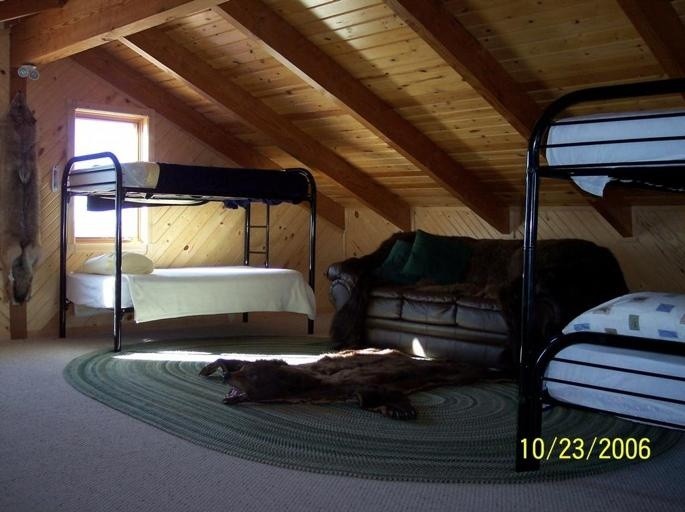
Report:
[56, 150, 320, 350]
[516, 78, 685, 474]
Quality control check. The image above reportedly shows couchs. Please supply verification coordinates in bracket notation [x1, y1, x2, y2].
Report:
[324, 226, 619, 377]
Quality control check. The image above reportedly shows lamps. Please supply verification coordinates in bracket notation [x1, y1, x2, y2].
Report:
[15, 64, 42, 81]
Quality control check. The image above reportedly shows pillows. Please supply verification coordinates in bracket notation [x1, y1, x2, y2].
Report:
[376, 227, 479, 287]
[78, 251, 155, 276]
[557, 291, 685, 345]
